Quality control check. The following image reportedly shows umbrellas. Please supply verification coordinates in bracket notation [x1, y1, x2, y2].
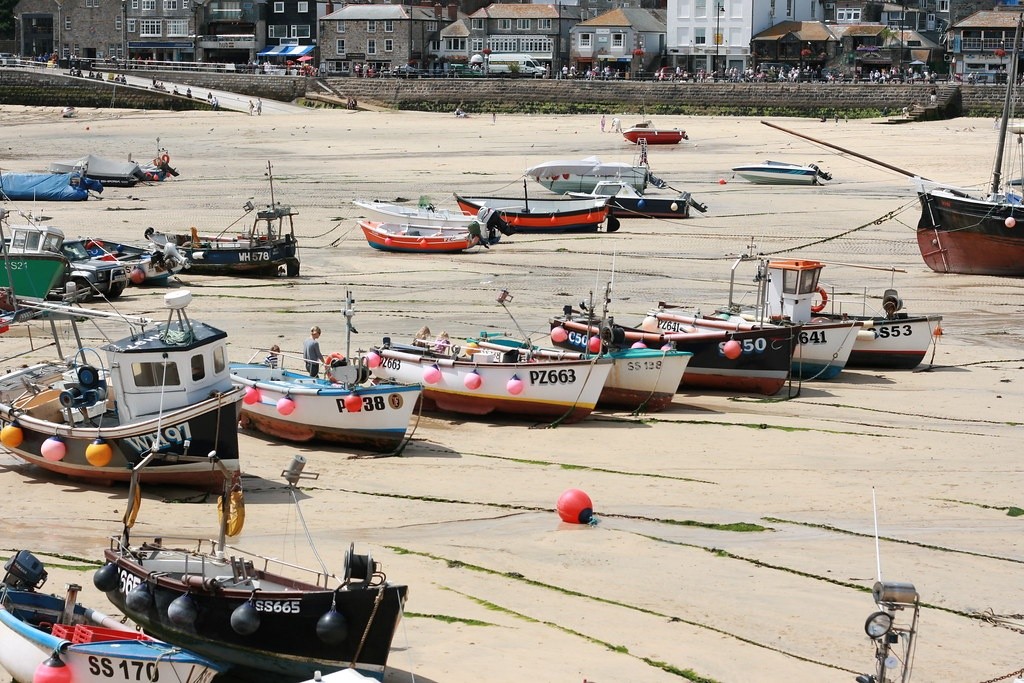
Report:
[910, 60, 925, 71]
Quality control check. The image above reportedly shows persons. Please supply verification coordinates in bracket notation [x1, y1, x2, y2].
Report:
[354, 62, 485, 79]
[562, 61, 938, 84]
[106, 55, 154, 70]
[968, 70, 980, 86]
[412, 326, 430, 350]
[115, 74, 127, 85]
[930, 87, 938, 105]
[262, 345, 280, 369]
[347, 95, 357, 110]
[193, 59, 319, 77]
[600, 114, 623, 133]
[639, 144, 650, 168]
[173, 86, 179, 95]
[207, 93, 219, 108]
[991, 68, 1024, 86]
[434, 332, 449, 354]
[152, 75, 167, 91]
[186, 88, 193, 99]
[303, 326, 326, 379]
[247, 98, 262, 116]
[68, 67, 103, 80]
[546, 63, 550, 78]
[12, 50, 77, 67]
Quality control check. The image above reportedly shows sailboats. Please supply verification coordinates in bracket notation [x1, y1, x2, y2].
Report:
[760, 11, 1024, 278]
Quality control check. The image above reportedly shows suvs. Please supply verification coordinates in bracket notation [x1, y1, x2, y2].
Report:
[756, 62, 793, 77]
[1, 237, 129, 301]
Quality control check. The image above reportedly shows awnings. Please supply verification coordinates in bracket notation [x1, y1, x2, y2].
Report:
[471, 54, 484, 62]
[255, 45, 317, 57]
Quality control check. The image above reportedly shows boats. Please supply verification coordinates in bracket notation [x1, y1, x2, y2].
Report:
[0, 551, 221, 682]
[0, 136, 302, 314]
[366, 242, 625, 425]
[94, 357, 409, 683]
[226, 290, 424, 454]
[349, 120, 833, 254]
[549, 235, 806, 396]
[465, 331, 696, 415]
[0, 289, 243, 497]
[645, 259, 944, 380]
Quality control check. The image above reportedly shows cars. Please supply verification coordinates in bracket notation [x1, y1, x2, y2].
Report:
[0, 53, 16, 67]
[655, 67, 691, 81]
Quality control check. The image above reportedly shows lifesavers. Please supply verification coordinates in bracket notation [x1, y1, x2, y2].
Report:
[217, 490, 246, 537]
[811, 286, 827, 312]
[325, 352, 345, 382]
[162, 154, 169, 163]
[86, 241, 104, 250]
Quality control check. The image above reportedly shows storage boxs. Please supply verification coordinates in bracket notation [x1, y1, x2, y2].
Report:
[51, 623, 151, 645]
[12, 367, 115, 424]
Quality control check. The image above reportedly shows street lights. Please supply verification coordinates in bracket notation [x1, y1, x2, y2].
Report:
[900, 3, 910, 70]
[558, 1, 567, 79]
[716, 2, 725, 76]
[405, 6, 413, 60]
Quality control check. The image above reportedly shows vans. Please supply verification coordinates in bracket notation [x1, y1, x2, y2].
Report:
[488, 54, 547, 79]
